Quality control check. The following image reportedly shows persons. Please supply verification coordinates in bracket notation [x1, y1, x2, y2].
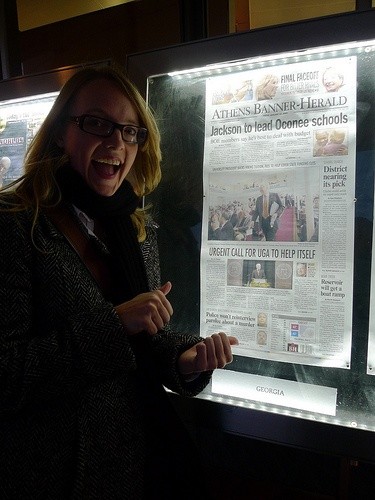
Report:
[258, 312, 268, 326]
[251, 263, 266, 279]
[0, 65, 239, 500]
[322, 66, 344, 92]
[0, 155, 11, 189]
[312, 129, 348, 157]
[322, 129, 347, 156]
[208, 176, 320, 243]
[257, 330, 267, 345]
[255, 72, 278, 101]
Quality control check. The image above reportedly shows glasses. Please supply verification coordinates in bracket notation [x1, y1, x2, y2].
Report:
[64, 113, 148, 145]
